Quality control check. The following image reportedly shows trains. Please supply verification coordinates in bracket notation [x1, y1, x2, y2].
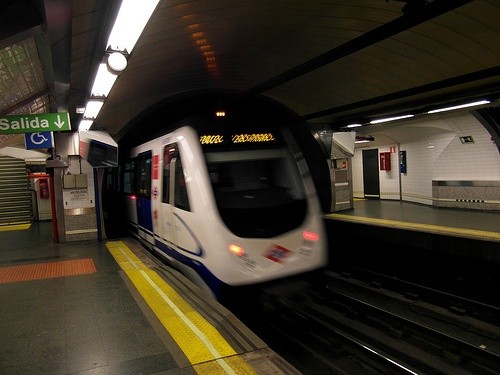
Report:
[105, 102, 328, 288]
